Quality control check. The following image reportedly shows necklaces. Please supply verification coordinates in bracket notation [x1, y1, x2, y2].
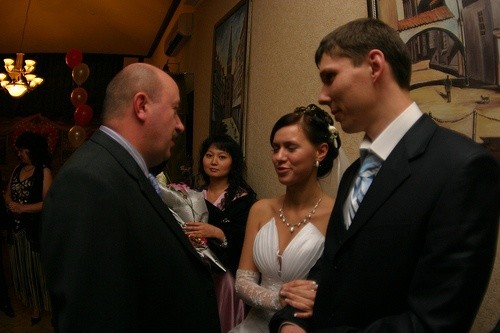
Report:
[280, 183, 322, 233]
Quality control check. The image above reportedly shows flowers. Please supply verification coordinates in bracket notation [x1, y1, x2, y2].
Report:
[329, 124, 338, 149]
[156, 171, 218, 260]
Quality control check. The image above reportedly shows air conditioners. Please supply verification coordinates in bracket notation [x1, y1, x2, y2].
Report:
[165, 13, 193, 57]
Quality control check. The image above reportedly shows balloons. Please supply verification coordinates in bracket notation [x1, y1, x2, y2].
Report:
[65, 47, 92, 149]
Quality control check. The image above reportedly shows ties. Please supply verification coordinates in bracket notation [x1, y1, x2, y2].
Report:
[343, 150, 381, 234]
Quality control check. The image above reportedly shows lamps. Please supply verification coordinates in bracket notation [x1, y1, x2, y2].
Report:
[1, 0, 44, 98]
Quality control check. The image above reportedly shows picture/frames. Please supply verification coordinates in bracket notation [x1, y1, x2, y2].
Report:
[209, 0, 252, 164]
[367, 0, 500, 150]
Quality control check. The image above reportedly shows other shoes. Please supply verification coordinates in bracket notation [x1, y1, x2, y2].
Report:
[31, 311, 41, 325]
[0, 303, 16, 317]
[149, 175, 169, 207]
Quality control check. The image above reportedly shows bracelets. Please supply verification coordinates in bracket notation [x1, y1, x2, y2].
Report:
[220, 237, 228, 248]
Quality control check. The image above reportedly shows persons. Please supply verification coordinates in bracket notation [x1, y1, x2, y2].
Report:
[35, 63, 222, 333]
[0, 190, 16, 318]
[181, 133, 261, 278]
[4, 133, 53, 325]
[268, 18, 500, 333]
[228, 103, 342, 333]
